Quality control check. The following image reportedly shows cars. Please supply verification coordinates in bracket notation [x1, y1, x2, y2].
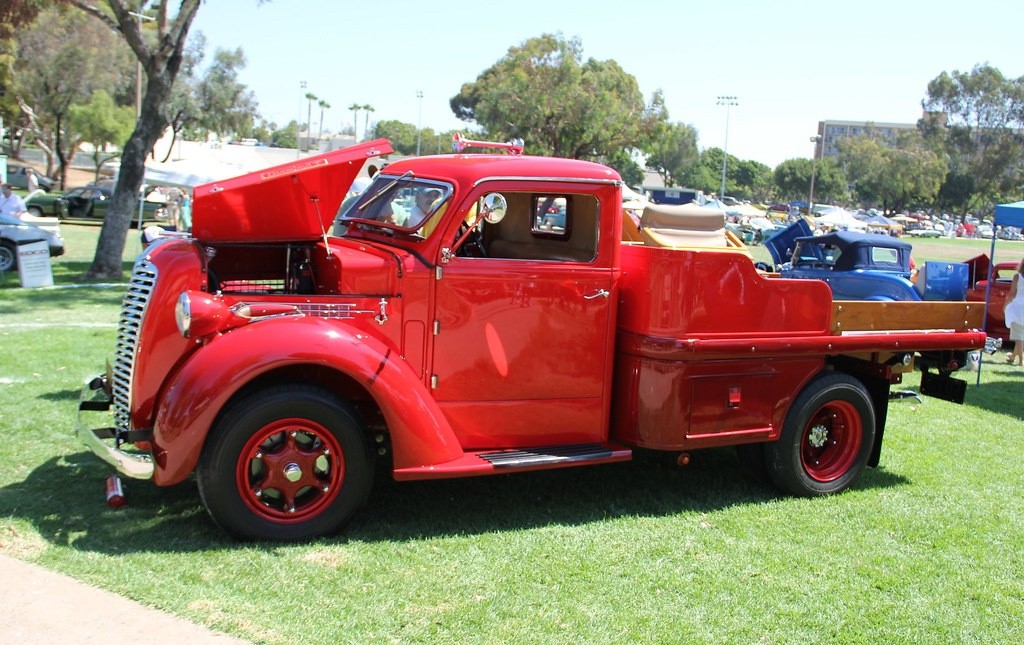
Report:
[956, 259, 1023, 342]
[714, 190, 1020, 246]
[0, 213, 64, 272]
[25, 182, 169, 224]
[5, 162, 55, 191]
[73, 123, 991, 547]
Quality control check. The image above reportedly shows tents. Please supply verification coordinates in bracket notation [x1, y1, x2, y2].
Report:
[976, 200, 1024, 387]
[138, 157, 267, 230]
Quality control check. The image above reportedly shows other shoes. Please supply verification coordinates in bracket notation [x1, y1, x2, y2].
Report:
[1017, 364, 1024, 367]
[1006, 352, 1015, 364]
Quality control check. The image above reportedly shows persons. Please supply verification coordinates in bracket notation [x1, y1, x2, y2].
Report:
[488, 206, 497, 221]
[1002, 257, 1024, 366]
[407, 186, 443, 233]
[0, 183, 27, 219]
[25, 168, 38, 192]
[178, 189, 193, 231]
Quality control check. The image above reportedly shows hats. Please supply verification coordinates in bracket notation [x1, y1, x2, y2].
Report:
[417, 185, 443, 194]
